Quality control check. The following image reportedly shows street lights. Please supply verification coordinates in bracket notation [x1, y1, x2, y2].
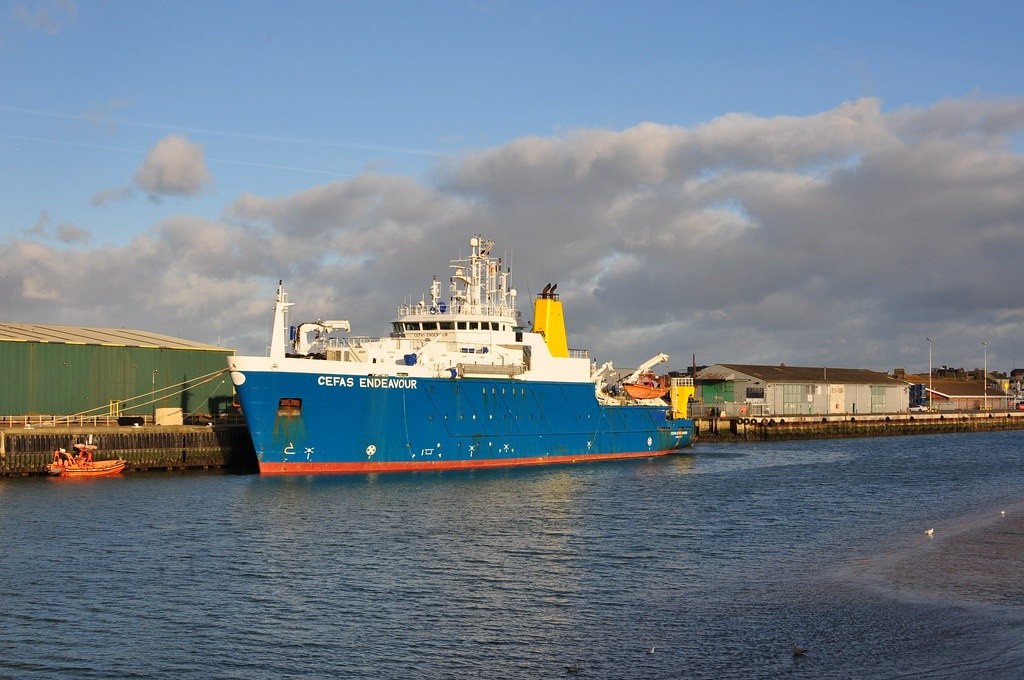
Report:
[981, 340, 991, 410]
[926, 336, 936, 412]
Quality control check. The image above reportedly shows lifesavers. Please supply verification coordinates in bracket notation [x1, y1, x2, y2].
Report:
[781, 419, 785, 425]
[822, 418, 827, 423]
[430, 307, 436, 314]
[940, 415, 945, 420]
[851, 418, 855, 423]
[751, 419, 757, 425]
[770, 419, 776, 426]
[886, 417, 890, 421]
[761, 419, 768, 426]
[911, 416, 914, 421]
[744, 418, 750, 425]
[737, 418, 743, 425]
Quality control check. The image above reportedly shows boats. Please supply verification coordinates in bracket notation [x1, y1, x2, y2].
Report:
[44, 438, 127, 477]
[225, 231, 696, 475]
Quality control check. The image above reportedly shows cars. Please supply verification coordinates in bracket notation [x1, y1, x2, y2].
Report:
[909, 404, 927, 412]
